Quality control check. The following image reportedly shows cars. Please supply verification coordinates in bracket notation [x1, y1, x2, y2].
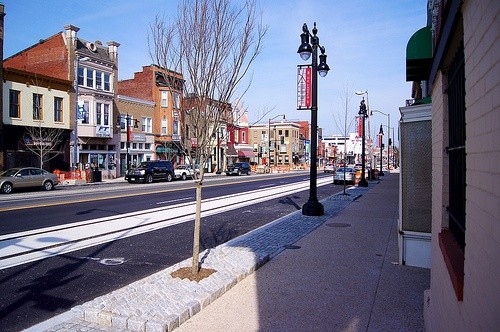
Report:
[174, 164, 202, 180]
[324, 164, 335, 173]
[354, 164, 366, 170]
[0, 167, 59, 193]
[333, 167, 356, 183]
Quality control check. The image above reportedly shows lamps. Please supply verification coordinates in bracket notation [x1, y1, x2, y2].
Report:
[358, 106, 365, 118]
[317, 46, 330, 77]
[297, 23, 313, 61]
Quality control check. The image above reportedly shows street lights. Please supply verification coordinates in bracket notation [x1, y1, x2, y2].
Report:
[358, 96, 370, 187]
[370, 110, 389, 173]
[297, 21, 330, 215]
[377, 124, 394, 167]
[116, 112, 139, 174]
[268, 114, 287, 166]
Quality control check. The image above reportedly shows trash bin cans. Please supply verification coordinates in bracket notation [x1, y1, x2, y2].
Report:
[367, 166, 375, 180]
[91, 163, 102, 182]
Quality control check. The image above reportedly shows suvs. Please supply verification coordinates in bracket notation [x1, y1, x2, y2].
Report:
[227, 162, 251, 176]
[125, 159, 173, 183]
